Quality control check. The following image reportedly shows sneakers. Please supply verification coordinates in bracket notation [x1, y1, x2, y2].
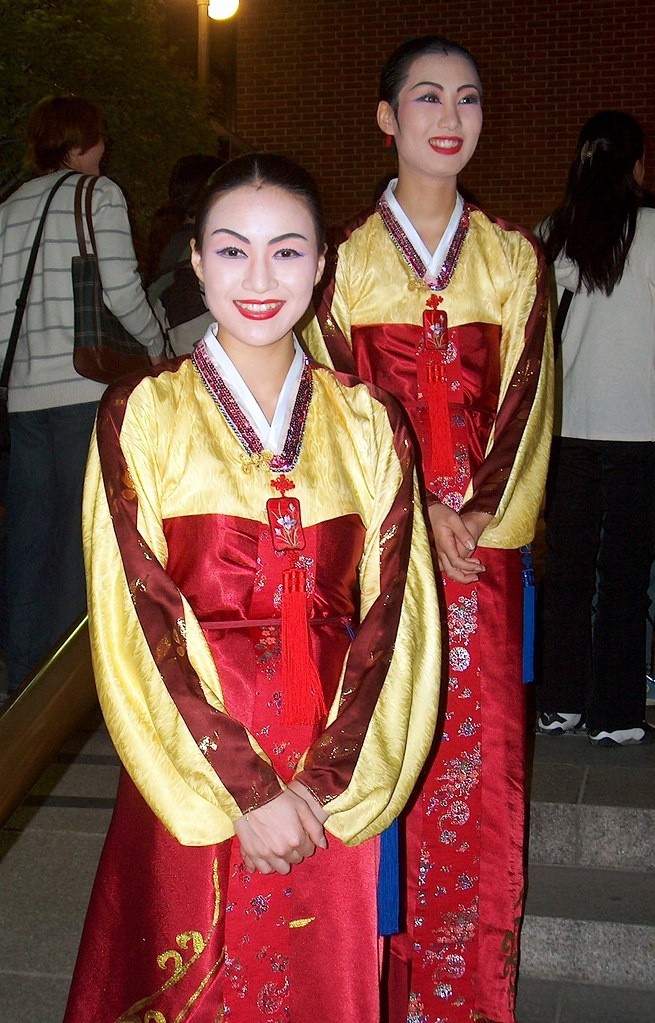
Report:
[525, 712, 587, 737]
[589, 720, 655, 747]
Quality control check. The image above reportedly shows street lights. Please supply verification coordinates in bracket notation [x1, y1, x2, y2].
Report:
[195, 0, 240, 79]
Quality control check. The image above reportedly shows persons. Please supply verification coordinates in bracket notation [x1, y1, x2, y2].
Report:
[531, 108, 654, 744]
[63, 152, 442, 1023]
[0, 95, 163, 697]
[291, 37, 554, 1023]
[147, 153, 215, 361]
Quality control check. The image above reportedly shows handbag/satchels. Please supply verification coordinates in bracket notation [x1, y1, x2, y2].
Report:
[0, 398, 11, 504]
[71, 176, 152, 385]
